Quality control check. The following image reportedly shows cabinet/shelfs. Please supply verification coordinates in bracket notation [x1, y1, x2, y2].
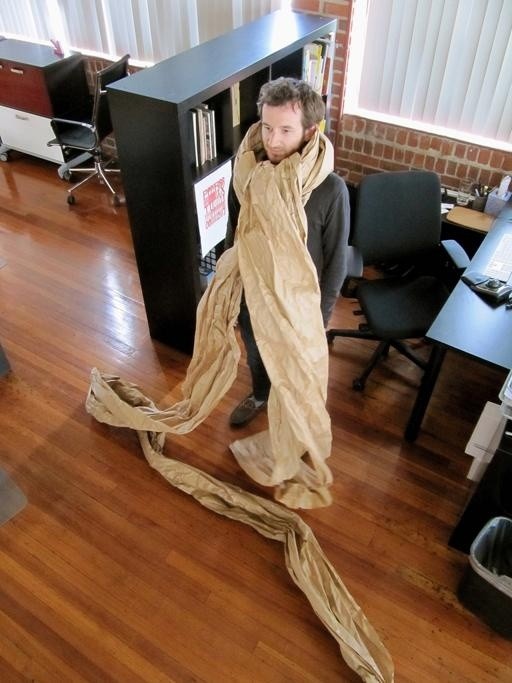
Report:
[0, 37, 84, 180]
[105, 8, 338, 359]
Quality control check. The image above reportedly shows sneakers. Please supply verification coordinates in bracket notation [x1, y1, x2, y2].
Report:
[225, 389, 267, 428]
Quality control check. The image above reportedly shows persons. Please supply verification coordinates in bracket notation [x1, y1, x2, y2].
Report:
[223, 76, 352, 427]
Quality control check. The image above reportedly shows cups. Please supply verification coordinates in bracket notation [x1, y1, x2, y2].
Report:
[471, 194, 485, 211]
[456, 177, 472, 207]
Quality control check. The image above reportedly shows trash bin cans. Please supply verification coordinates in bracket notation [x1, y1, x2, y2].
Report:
[461, 516, 512, 640]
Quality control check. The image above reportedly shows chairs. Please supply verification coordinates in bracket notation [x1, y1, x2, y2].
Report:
[326, 169, 470, 392]
[46, 52, 130, 206]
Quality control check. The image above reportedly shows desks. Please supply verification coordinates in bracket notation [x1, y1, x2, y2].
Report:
[404, 196, 512, 442]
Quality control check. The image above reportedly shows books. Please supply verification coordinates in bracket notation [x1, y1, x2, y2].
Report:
[302, 32, 333, 96]
[188, 103, 217, 168]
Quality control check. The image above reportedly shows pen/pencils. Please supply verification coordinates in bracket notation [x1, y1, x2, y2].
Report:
[474, 185, 488, 198]
